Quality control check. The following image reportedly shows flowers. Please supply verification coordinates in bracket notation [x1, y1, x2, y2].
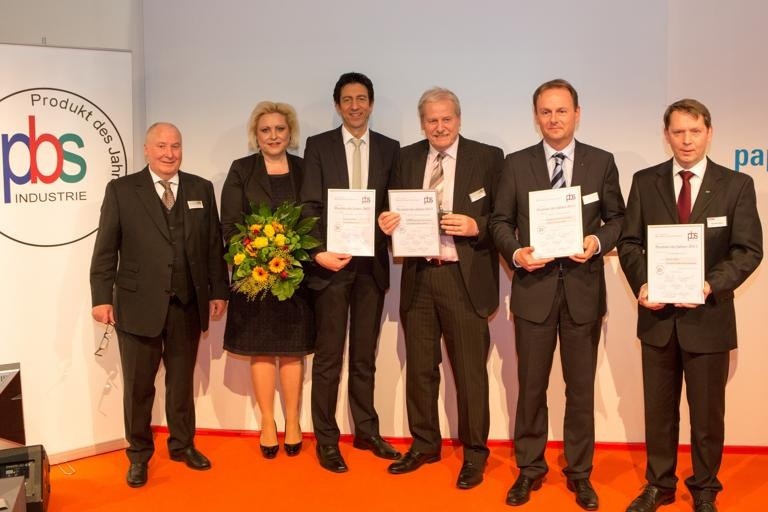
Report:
[224, 195, 324, 303]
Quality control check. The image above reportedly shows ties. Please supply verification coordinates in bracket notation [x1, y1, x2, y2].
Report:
[677, 170, 691, 224]
[158, 180, 176, 211]
[350, 139, 363, 189]
[551, 153, 566, 189]
[425, 152, 444, 262]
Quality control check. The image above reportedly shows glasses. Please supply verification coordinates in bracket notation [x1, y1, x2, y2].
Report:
[94, 320, 115, 356]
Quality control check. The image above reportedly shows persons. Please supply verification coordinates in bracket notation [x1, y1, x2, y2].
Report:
[220, 102, 315, 461]
[90, 123, 228, 488]
[299, 72, 402, 472]
[487, 79, 626, 511]
[617, 99, 764, 512]
[375, 88, 505, 489]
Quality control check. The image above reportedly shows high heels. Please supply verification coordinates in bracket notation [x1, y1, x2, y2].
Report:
[284, 424, 302, 457]
[260, 420, 279, 459]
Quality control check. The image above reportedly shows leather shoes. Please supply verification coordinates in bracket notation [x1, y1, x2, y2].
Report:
[388, 451, 441, 473]
[456, 465, 483, 488]
[506, 474, 542, 506]
[126, 462, 147, 488]
[566, 478, 599, 510]
[625, 482, 676, 512]
[316, 444, 348, 473]
[353, 434, 401, 460]
[169, 448, 211, 470]
[694, 501, 716, 511]
